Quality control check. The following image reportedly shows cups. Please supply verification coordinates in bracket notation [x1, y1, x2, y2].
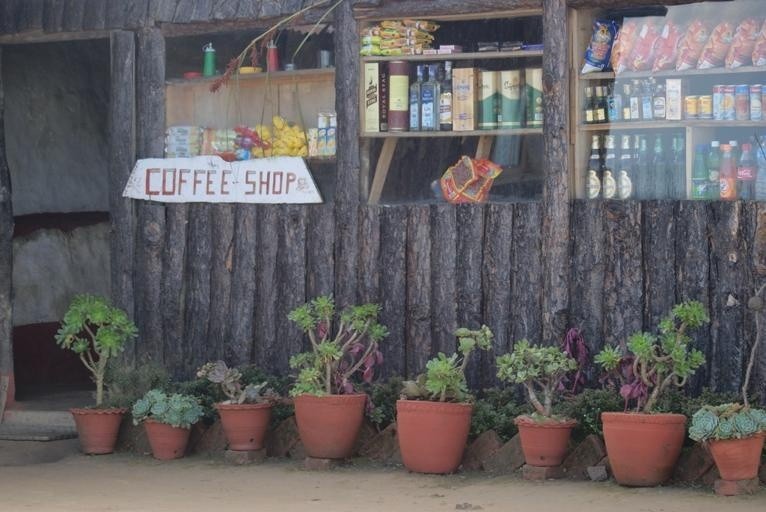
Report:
[316, 50, 331, 68]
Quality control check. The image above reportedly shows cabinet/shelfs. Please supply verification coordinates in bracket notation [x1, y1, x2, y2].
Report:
[165, 66, 336, 169]
[360, 47, 548, 134]
[572, 1, 766, 199]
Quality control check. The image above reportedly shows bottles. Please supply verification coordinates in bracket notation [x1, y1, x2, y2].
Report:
[267, 40, 280, 73]
[585, 129, 766, 200]
[409, 60, 454, 132]
[201, 42, 216, 76]
[584, 77, 666, 122]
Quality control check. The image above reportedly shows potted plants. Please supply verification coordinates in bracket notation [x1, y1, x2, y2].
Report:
[592, 298, 714, 488]
[495, 338, 582, 467]
[195, 358, 282, 450]
[688, 400, 766, 480]
[283, 290, 391, 459]
[45, 294, 140, 455]
[126, 385, 205, 458]
[389, 322, 494, 479]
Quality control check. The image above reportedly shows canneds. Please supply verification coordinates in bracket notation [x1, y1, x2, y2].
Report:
[681, 83, 766, 122]
